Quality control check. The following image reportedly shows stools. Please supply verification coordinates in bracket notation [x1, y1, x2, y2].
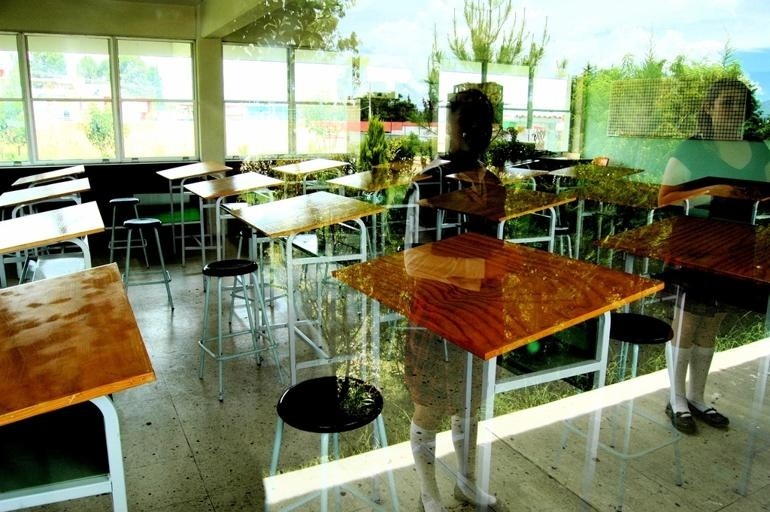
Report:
[263, 374, 401, 512]
[197, 258, 285, 403]
[108, 196, 152, 270]
[122, 217, 175, 312]
[559, 312, 685, 507]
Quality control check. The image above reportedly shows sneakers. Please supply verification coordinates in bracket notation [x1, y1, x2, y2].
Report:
[454, 485, 498, 508]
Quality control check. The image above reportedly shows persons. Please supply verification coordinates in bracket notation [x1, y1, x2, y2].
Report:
[655, 78, 770, 432]
[403, 89, 510, 512]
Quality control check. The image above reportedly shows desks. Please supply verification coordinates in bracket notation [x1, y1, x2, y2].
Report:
[324, 168, 433, 245]
[270, 158, 350, 200]
[676, 175, 769, 225]
[227, 190, 387, 386]
[183, 169, 285, 306]
[1, 177, 91, 220]
[331, 230, 665, 512]
[11, 163, 85, 183]
[594, 213, 770, 500]
[155, 160, 234, 266]
[0, 260, 159, 512]
[416, 184, 577, 259]
[547, 163, 646, 183]
[446, 166, 550, 188]
[0, 199, 106, 287]
[560, 180, 690, 276]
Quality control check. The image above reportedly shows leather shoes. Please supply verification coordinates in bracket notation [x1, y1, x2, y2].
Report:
[687, 400, 729, 428]
[665, 402, 696, 434]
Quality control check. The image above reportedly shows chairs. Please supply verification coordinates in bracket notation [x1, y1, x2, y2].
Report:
[591, 155, 610, 166]
